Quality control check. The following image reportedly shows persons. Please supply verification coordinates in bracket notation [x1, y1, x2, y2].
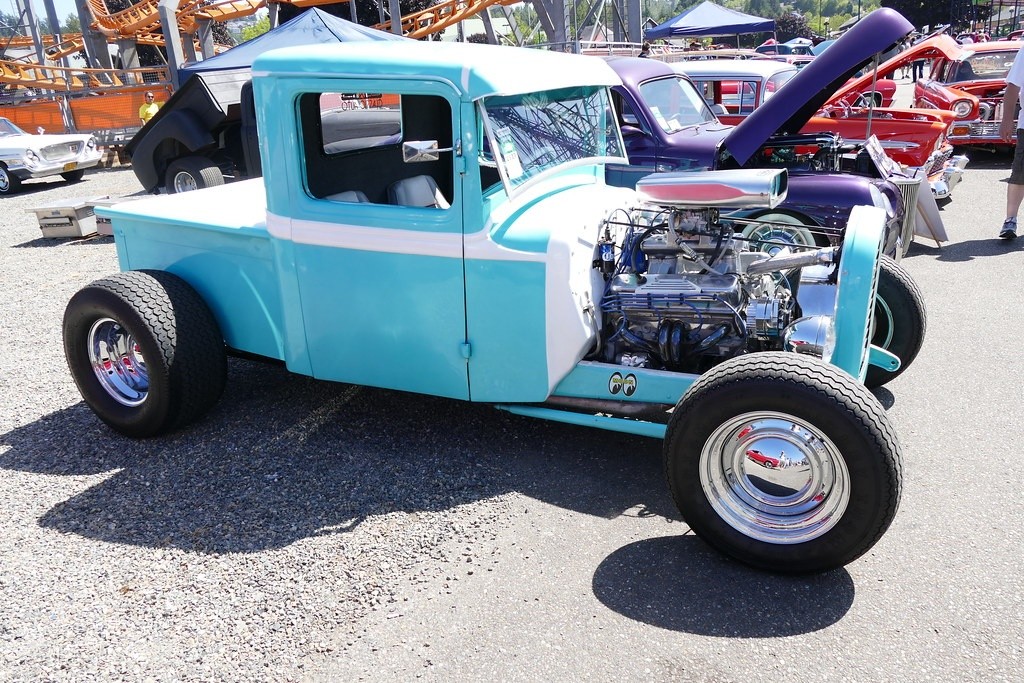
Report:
[998, 44, 1024, 240]
[980, 35, 987, 42]
[138, 90, 159, 128]
[962, 34, 973, 44]
[902, 35, 925, 84]
[952, 33, 963, 44]
[638, 42, 651, 58]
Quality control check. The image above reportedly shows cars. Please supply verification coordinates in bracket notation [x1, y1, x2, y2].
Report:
[0, 116, 104, 195]
[62, 39, 929, 580]
[117, 0, 1024, 270]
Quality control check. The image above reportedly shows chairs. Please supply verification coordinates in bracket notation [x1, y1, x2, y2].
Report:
[387, 174, 449, 211]
[319, 189, 372, 203]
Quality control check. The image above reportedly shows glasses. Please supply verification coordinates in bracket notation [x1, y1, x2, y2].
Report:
[148, 96, 154, 99]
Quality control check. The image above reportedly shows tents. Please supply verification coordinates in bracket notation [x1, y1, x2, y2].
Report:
[643, 1, 779, 56]
[177, 6, 421, 116]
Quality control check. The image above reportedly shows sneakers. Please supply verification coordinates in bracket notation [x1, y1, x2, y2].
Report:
[999, 217, 1018, 239]
